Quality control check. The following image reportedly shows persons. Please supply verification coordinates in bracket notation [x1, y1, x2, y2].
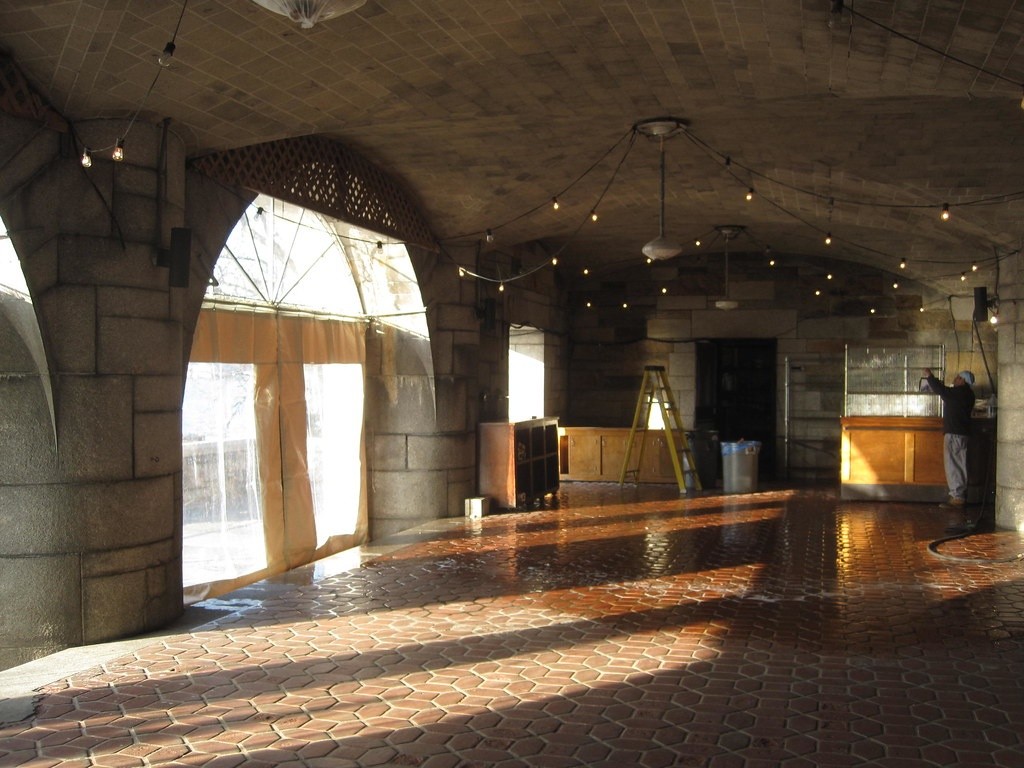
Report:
[922, 368, 975, 508]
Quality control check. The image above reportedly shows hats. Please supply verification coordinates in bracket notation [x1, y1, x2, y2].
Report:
[960, 370, 974, 386]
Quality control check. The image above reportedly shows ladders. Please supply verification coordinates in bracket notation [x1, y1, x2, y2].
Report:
[621, 365, 703, 494]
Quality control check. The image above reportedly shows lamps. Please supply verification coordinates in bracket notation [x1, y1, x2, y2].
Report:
[251, 0, 367, 28]
[973, 285, 996, 323]
[153, 226, 191, 288]
[642, 123, 685, 261]
[712, 225, 744, 310]
[477, 296, 497, 331]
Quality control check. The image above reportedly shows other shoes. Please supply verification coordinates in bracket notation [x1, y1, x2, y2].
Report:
[939, 497, 965, 509]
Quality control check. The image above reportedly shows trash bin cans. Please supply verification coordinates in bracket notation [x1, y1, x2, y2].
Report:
[719, 440, 762, 493]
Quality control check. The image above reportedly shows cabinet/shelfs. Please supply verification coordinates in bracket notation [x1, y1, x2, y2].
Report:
[782, 356, 839, 478]
[478, 414, 560, 511]
[556, 427, 683, 484]
[844, 341, 946, 417]
